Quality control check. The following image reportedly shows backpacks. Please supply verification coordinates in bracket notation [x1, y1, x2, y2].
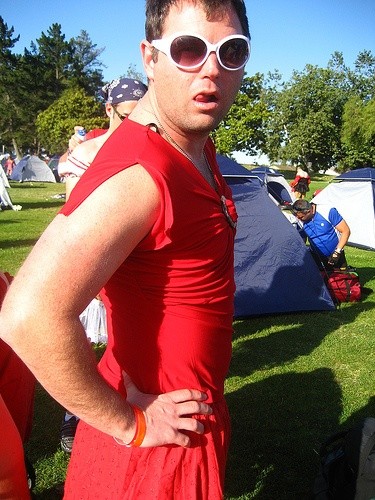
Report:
[295, 182, 309, 193]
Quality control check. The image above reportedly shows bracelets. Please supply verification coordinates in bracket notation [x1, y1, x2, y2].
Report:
[113, 400, 147, 447]
[336, 247, 343, 252]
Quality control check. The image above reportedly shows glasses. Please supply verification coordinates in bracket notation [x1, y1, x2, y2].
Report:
[291, 205, 307, 211]
[110, 103, 125, 122]
[151, 35, 253, 71]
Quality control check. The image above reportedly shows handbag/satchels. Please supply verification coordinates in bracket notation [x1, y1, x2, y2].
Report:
[321, 267, 361, 302]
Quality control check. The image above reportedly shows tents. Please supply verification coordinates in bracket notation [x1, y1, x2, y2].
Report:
[0, 152, 60, 211]
[310, 167, 375, 252]
[250, 166, 297, 209]
[213, 152, 337, 315]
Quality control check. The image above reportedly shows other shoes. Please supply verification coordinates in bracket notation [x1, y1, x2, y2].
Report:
[60, 415, 79, 453]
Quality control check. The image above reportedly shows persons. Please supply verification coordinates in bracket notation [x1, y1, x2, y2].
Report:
[5, 156, 17, 175]
[0, 0, 252, 500]
[292, 199, 351, 267]
[289, 165, 311, 199]
[59, 78, 149, 452]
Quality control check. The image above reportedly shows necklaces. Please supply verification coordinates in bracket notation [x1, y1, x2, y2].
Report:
[138, 99, 215, 183]
